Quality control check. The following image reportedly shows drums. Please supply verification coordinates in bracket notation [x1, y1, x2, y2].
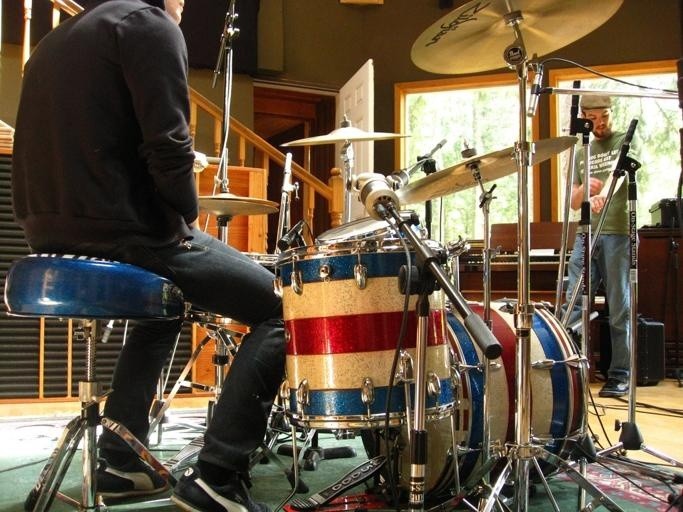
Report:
[379, 299, 581, 501]
[315, 211, 418, 242]
[272, 237, 455, 428]
[184, 252, 279, 325]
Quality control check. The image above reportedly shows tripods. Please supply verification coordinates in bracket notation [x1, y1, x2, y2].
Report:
[441, 1, 624, 508]
[573, 120, 682, 469]
[142, 0, 307, 492]
[374, 203, 501, 503]
[536, 77, 683, 507]
[254, 151, 353, 477]
[401, 139, 455, 301]
[537, 85, 683, 102]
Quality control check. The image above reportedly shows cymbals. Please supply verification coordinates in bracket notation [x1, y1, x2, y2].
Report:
[198, 193, 279, 217]
[395, 135, 578, 204]
[411, 2, 623, 75]
[280, 126, 410, 149]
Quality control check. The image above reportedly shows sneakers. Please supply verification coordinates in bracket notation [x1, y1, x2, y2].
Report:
[96, 458, 168, 497]
[171, 464, 271, 512]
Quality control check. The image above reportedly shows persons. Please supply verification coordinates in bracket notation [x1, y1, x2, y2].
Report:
[14, 0, 286, 512]
[558, 91, 643, 398]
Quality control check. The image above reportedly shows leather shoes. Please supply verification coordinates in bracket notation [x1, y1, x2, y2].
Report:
[599, 379, 629, 396]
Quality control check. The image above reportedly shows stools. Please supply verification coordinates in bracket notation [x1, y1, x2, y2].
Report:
[3, 253, 185, 512]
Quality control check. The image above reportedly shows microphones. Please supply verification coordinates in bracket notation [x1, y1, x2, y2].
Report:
[279, 220, 304, 248]
[527, 63, 545, 115]
[388, 169, 413, 190]
[359, 177, 401, 221]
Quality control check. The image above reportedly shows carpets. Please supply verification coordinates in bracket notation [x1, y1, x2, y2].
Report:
[1, 409, 682, 512]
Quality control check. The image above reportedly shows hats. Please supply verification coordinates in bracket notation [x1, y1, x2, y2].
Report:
[581, 95, 611, 108]
